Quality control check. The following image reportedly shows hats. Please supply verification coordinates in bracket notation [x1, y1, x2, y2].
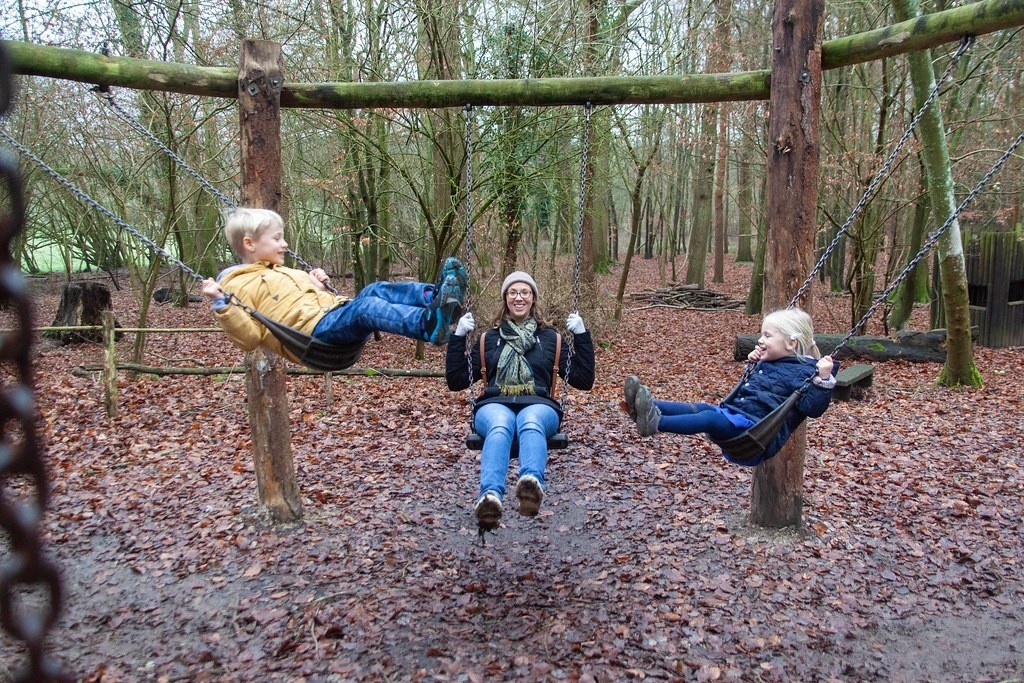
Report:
[501, 271, 538, 301]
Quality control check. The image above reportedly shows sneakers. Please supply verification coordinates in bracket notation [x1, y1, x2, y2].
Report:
[472, 490, 503, 532]
[516, 475, 544, 517]
[423, 256, 467, 346]
[624, 376, 662, 437]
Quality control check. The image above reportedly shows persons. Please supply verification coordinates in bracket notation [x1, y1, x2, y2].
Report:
[445, 270, 595, 528]
[624, 307, 841, 467]
[201, 208, 467, 370]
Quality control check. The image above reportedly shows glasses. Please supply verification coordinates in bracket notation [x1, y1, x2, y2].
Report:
[505, 291, 531, 299]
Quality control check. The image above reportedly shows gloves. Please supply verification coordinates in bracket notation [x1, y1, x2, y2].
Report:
[566, 311, 586, 334]
[454, 312, 475, 336]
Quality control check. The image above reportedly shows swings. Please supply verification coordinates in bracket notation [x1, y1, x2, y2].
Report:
[708, 28, 1017, 462]
[0, 85, 374, 371]
[459, 100, 595, 450]
